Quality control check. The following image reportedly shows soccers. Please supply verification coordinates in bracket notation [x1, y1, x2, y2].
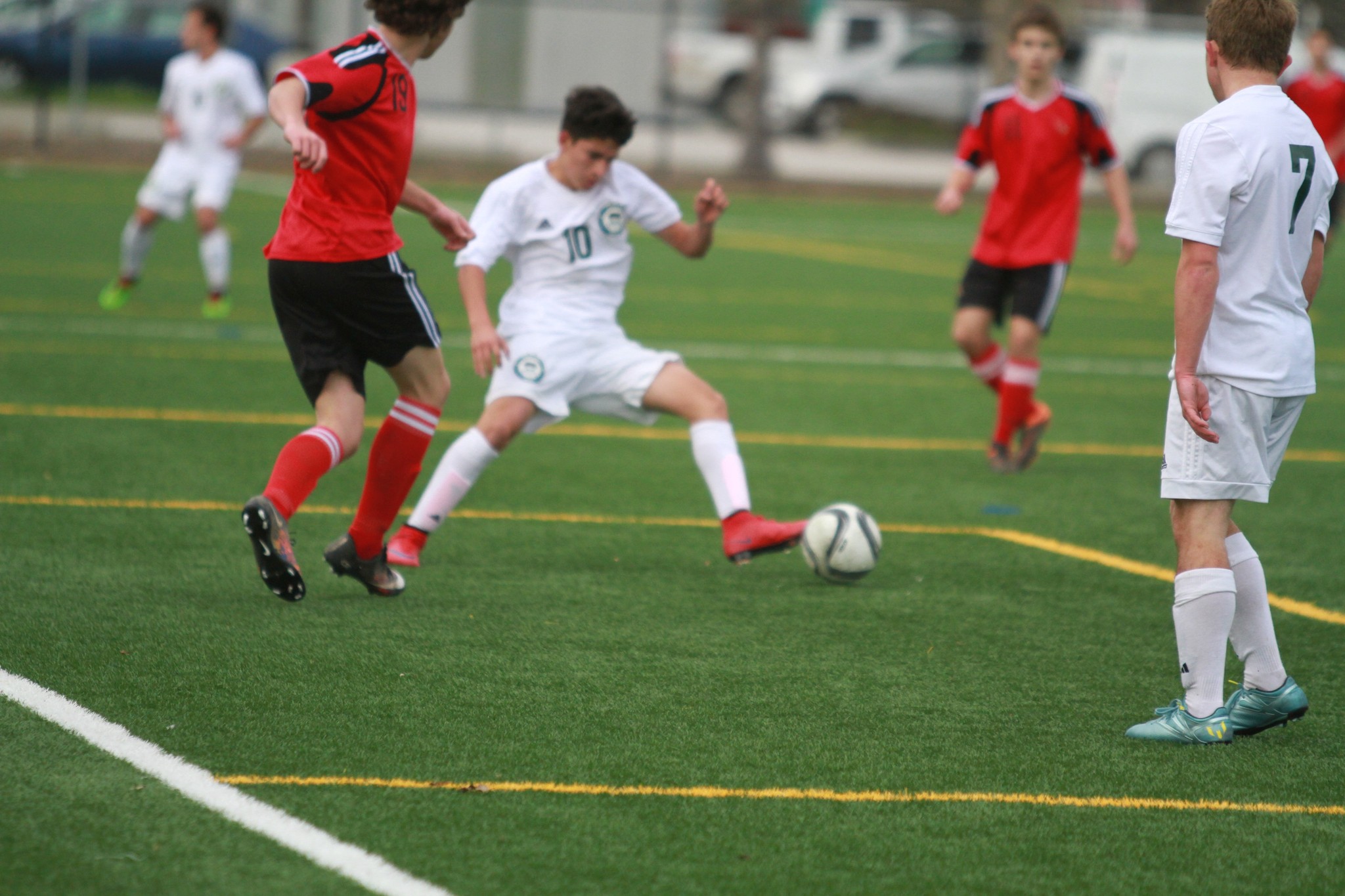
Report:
[798, 501, 883, 585]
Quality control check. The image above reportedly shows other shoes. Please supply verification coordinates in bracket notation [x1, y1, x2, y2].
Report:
[201, 286, 234, 320]
[97, 278, 131, 312]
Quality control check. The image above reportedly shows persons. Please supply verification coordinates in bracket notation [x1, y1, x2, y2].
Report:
[1125, 0, 1338, 745]
[242, 0, 476, 601]
[1274, 18, 1345, 255]
[385, 85, 809, 566]
[931, 6, 1138, 475]
[97, 0, 267, 320]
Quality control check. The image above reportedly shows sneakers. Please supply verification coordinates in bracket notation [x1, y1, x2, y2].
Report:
[985, 442, 1013, 472]
[322, 532, 406, 596]
[240, 496, 306, 603]
[1011, 402, 1049, 470]
[1224, 676, 1308, 740]
[1125, 699, 1232, 746]
[384, 524, 429, 568]
[720, 510, 811, 566]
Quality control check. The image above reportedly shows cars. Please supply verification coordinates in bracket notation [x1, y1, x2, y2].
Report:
[0, 1, 314, 101]
[654, 0, 958, 141]
[788, 39, 1070, 154]
